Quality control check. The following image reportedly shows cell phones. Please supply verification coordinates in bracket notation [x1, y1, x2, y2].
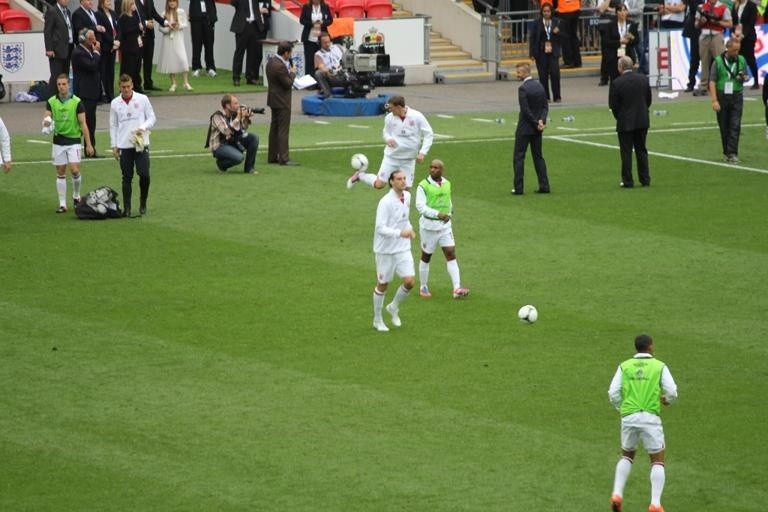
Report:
[739, 71, 743, 78]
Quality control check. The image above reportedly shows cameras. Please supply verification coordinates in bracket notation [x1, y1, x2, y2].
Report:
[247, 107, 265, 114]
[702, 11, 720, 21]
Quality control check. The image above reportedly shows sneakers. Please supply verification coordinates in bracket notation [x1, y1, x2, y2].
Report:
[420, 286, 431, 297]
[373, 319, 388, 331]
[347, 172, 360, 188]
[453, 288, 469, 298]
[649, 503, 665, 512]
[611, 494, 623, 512]
[387, 304, 401, 326]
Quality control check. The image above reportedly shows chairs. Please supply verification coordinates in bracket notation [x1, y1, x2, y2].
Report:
[275, 0, 392, 19]
[0, 0, 32, 32]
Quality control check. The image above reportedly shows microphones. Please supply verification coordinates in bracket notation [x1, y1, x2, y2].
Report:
[288, 58, 293, 67]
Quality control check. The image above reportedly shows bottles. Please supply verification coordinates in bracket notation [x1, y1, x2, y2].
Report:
[494, 117, 504, 124]
[439, 210, 454, 225]
[652, 109, 671, 116]
[561, 116, 575, 122]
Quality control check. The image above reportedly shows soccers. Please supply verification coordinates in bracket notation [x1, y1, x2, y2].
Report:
[518, 305, 537, 323]
[351, 152, 369, 171]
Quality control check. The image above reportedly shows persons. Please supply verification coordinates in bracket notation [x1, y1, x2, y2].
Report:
[156, 1, 194, 92]
[42, 1, 177, 104]
[610, 56, 652, 186]
[43, 73, 94, 213]
[583, 1, 649, 87]
[763, 73, 768, 127]
[510, 62, 551, 194]
[651, 1, 768, 30]
[71, 28, 106, 158]
[313, 32, 352, 100]
[372, 170, 417, 332]
[205, 93, 258, 174]
[109, 74, 156, 218]
[188, 1, 218, 77]
[472, 1, 498, 15]
[709, 38, 750, 162]
[529, 0, 581, 102]
[300, 1, 333, 90]
[682, 2, 759, 96]
[266, 41, 301, 165]
[0, 117, 12, 173]
[229, 1, 272, 86]
[347, 96, 433, 191]
[415, 159, 471, 299]
[608, 334, 678, 512]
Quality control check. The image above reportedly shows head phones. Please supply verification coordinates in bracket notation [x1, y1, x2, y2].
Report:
[724, 53, 738, 65]
[78, 27, 88, 43]
[317, 33, 329, 46]
